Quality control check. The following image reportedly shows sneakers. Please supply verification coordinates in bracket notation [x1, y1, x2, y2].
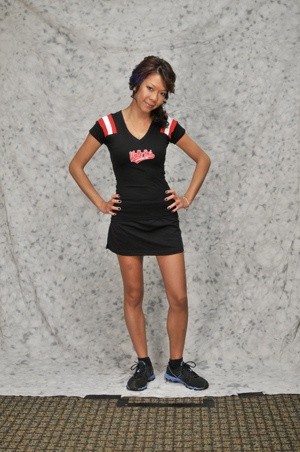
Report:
[164, 360, 209, 391]
[126, 360, 155, 391]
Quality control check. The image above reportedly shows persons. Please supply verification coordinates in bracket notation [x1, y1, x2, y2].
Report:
[69, 54, 212, 390]
